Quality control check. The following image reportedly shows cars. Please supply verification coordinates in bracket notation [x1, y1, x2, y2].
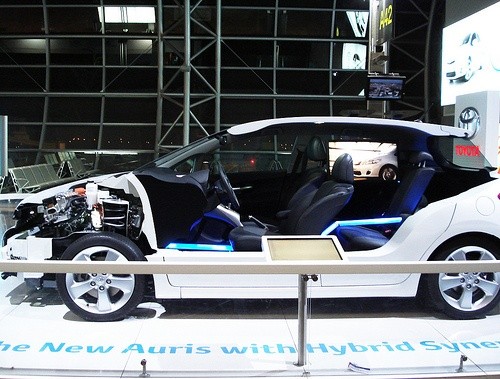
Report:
[352, 146, 398, 180]
[1, 116, 500, 321]
[445, 31, 484, 84]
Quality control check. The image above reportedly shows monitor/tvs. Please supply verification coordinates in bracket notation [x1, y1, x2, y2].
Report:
[365, 74, 406, 101]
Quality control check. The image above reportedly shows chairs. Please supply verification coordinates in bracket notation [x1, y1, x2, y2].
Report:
[275, 136, 329, 223]
[228, 156, 355, 251]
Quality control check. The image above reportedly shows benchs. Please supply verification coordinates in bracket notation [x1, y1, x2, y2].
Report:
[335, 151, 436, 250]
[7, 159, 99, 193]
